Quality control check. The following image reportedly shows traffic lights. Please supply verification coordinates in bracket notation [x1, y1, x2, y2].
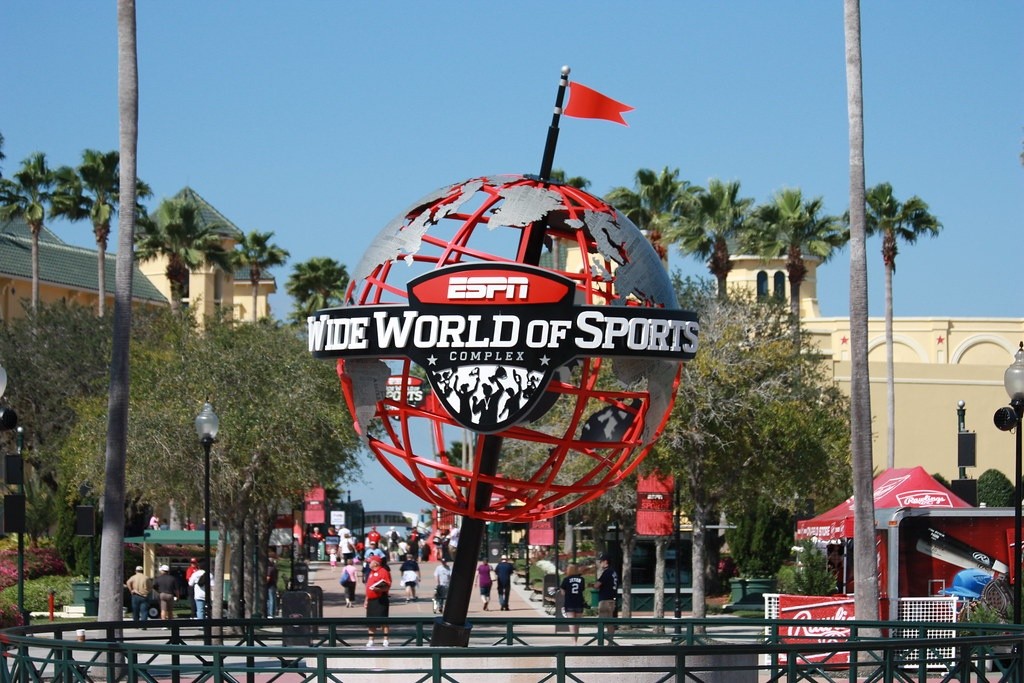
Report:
[0, 451, 27, 535]
[0, 408, 19, 432]
[956, 431, 981, 474]
[74, 505, 95, 538]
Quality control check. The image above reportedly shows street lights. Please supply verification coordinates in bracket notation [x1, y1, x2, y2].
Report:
[192, 401, 218, 649]
[486, 519, 491, 567]
[991, 341, 1023, 665]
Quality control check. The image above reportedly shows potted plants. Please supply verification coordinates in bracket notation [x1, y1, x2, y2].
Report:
[724, 492, 796, 606]
[54, 471, 102, 616]
[957, 603, 1002, 672]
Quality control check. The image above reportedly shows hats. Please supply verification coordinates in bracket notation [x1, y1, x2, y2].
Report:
[136, 566, 142, 571]
[498, 554, 507, 559]
[441, 558, 447, 562]
[159, 565, 169, 572]
[598, 553, 612, 562]
[190, 559, 198, 564]
[366, 555, 381, 564]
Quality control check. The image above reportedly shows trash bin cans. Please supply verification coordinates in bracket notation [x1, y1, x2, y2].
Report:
[293, 562, 308, 588]
[307, 586, 324, 618]
[542, 574, 560, 606]
[280, 591, 314, 648]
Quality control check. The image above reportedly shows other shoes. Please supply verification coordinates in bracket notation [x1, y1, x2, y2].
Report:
[501, 603, 510, 611]
[483, 601, 488, 612]
[383, 639, 389, 648]
[367, 638, 373, 647]
[406, 595, 418, 603]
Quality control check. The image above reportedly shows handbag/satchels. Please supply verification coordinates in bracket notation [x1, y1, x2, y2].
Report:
[341, 565, 349, 587]
[198, 574, 207, 587]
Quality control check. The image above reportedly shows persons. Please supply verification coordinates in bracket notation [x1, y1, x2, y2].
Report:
[152, 565, 178, 631]
[434, 559, 452, 613]
[184, 517, 205, 531]
[267, 558, 278, 618]
[364, 556, 392, 647]
[494, 555, 513, 611]
[400, 555, 422, 603]
[186, 558, 214, 619]
[150, 513, 168, 530]
[341, 560, 358, 608]
[594, 554, 618, 644]
[560, 564, 585, 641]
[474, 559, 493, 611]
[311, 525, 458, 598]
[127, 566, 150, 630]
